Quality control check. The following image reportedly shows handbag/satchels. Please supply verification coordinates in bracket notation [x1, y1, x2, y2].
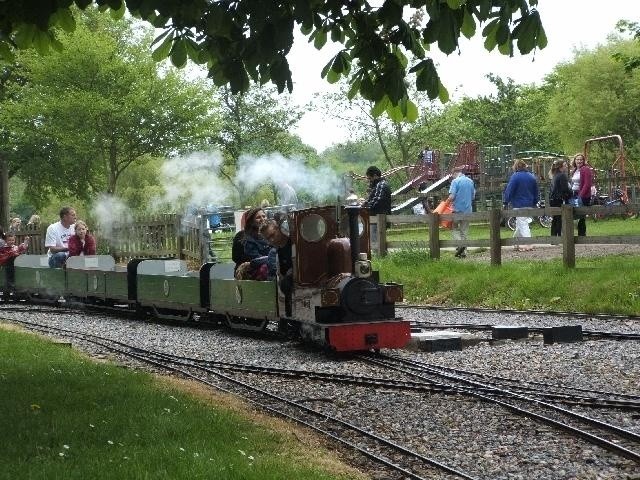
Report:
[432, 201, 453, 230]
[566, 190, 586, 221]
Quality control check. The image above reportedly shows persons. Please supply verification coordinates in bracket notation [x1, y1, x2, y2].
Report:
[27, 214, 41, 231]
[359, 165, 391, 256]
[0, 232, 31, 267]
[10, 218, 21, 231]
[444, 166, 477, 258]
[346, 188, 359, 206]
[548, 160, 572, 245]
[277, 178, 300, 205]
[570, 153, 593, 236]
[44, 208, 76, 268]
[231, 208, 275, 281]
[501, 159, 540, 251]
[69, 220, 95, 256]
[262, 222, 293, 298]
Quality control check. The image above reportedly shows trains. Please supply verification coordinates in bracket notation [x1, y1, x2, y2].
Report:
[1, 203, 414, 356]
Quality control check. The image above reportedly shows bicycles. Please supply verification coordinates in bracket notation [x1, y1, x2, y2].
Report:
[507, 203, 553, 231]
[592, 185, 637, 223]
